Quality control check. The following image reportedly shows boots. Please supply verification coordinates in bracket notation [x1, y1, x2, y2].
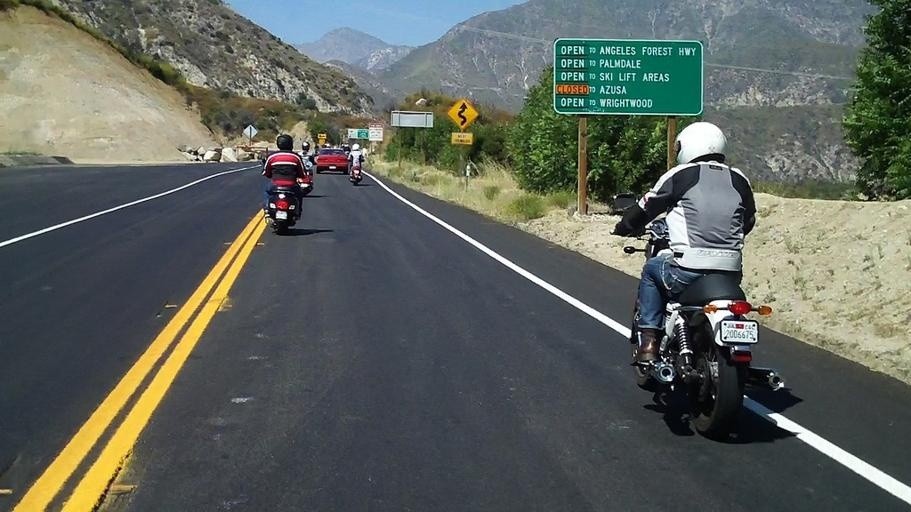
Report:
[634, 327, 664, 362]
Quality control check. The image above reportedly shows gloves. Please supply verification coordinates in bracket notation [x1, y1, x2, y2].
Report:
[615, 221, 645, 237]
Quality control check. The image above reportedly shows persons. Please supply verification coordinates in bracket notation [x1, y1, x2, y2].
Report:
[613, 121, 756, 366]
[260, 133, 308, 219]
[295, 139, 318, 176]
[347, 143, 365, 178]
[313, 142, 351, 157]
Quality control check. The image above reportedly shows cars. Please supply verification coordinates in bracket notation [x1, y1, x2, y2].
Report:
[316, 148, 349, 173]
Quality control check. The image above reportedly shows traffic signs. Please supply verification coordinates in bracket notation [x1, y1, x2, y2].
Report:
[358, 129, 368, 138]
[554, 37, 704, 117]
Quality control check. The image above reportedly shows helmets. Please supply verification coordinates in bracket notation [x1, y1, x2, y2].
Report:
[352, 143, 359, 150]
[302, 142, 310, 150]
[277, 135, 293, 150]
[674, 121, 727, 164]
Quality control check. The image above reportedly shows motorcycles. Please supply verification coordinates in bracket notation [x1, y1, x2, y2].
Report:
[347, 159, 366, 186]
[296, 169, 314, 195]
[611, 190, 785, 439]
[261, 161, 313, 235]
